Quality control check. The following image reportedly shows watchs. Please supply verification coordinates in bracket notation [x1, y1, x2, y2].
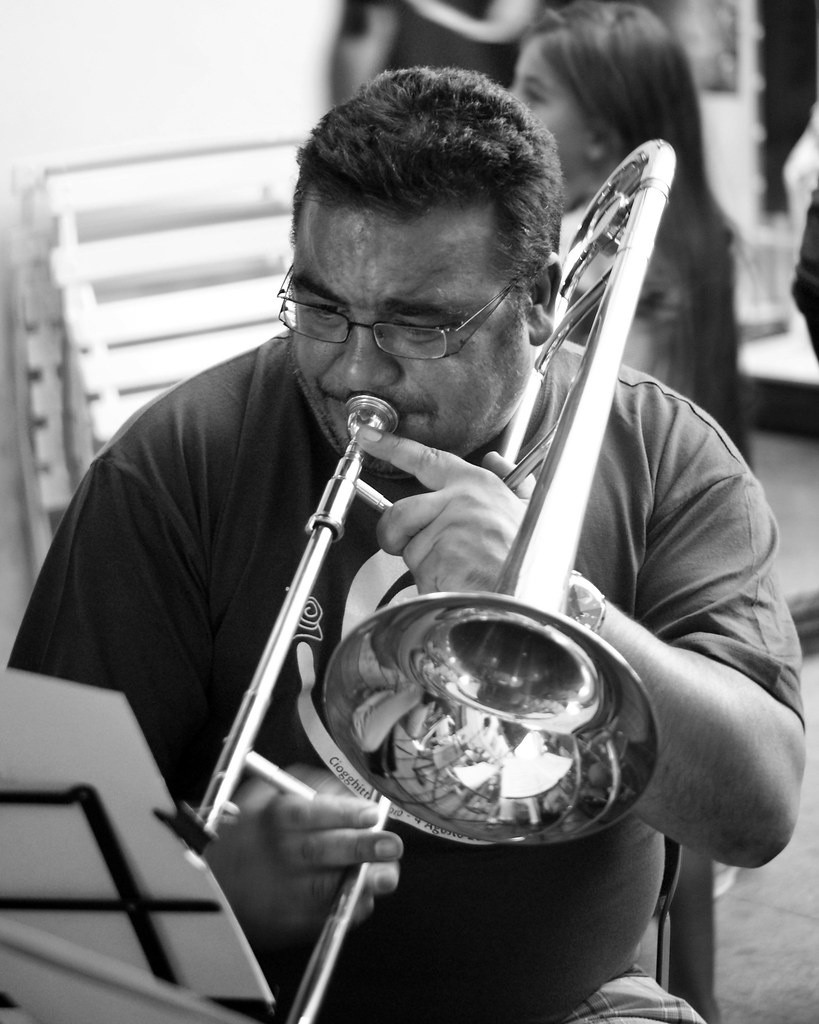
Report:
[563, 570, 606, 635]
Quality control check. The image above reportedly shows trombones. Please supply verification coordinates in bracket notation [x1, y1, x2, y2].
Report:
[179, 134, 680, 1024]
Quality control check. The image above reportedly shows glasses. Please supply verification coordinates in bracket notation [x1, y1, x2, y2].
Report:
[277, 263, 526, 361]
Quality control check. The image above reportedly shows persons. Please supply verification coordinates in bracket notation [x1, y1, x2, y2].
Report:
[510, 4, 737, 917]
[11, 68, 804, 1024]
[328, 0, 819, 368]
